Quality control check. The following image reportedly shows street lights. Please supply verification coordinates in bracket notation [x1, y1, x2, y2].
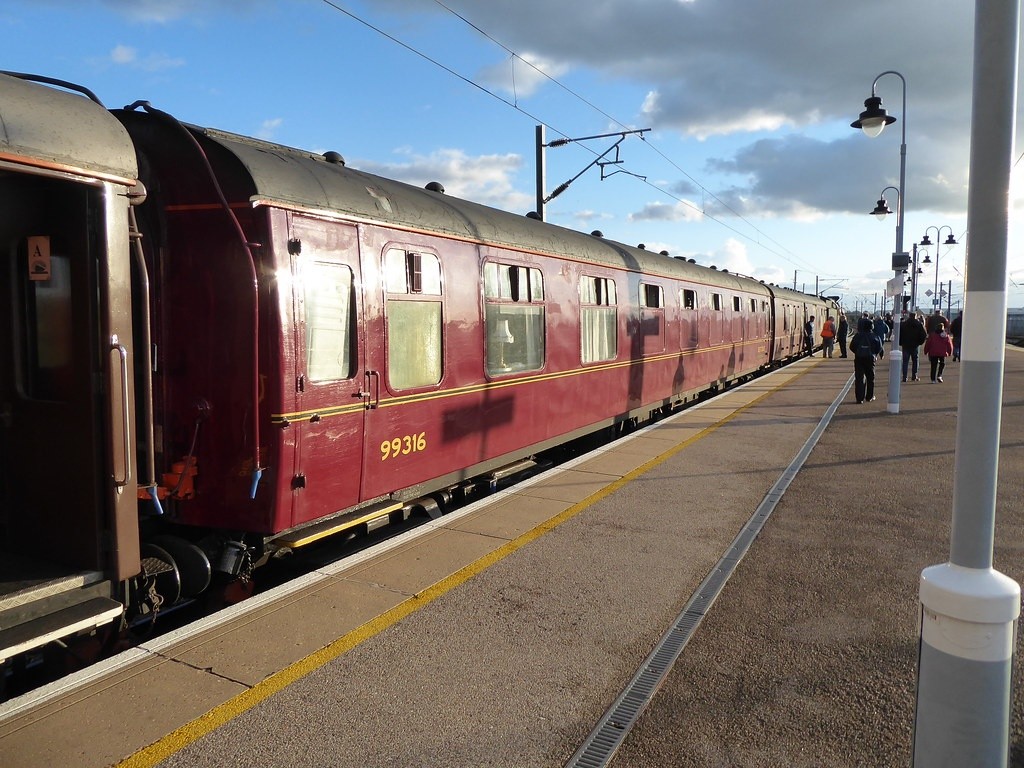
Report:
[850, 71, 958, 414]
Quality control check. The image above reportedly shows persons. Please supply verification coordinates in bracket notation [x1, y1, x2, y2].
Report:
[849, 318, 876, 403]
[924, 322, 952, 384]
[837, 316, 848, 358]
[927, 309, 947, 333]
[858, 312, 874, 332]
[876, 316, 888, 358]
[804, 315, 815, 358]
[900, 313, 920, 382]
[952, 310, 963, 361]
[820, 316, 836, 358]
[871, 312, 930, 339]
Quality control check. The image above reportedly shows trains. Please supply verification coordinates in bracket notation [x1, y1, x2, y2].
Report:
[0, 65, 849, 687]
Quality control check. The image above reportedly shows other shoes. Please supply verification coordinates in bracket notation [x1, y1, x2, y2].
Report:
[937, 377, 944, 383]
[857, 396, 876, 403]
[911, 377, 920, 381]
[811, 356, 847, 358]
[876, 356, 884, 360]
[903, 376, 908, 382]
[953, 357, 960, 361]
[932, 381, 938, 384]
